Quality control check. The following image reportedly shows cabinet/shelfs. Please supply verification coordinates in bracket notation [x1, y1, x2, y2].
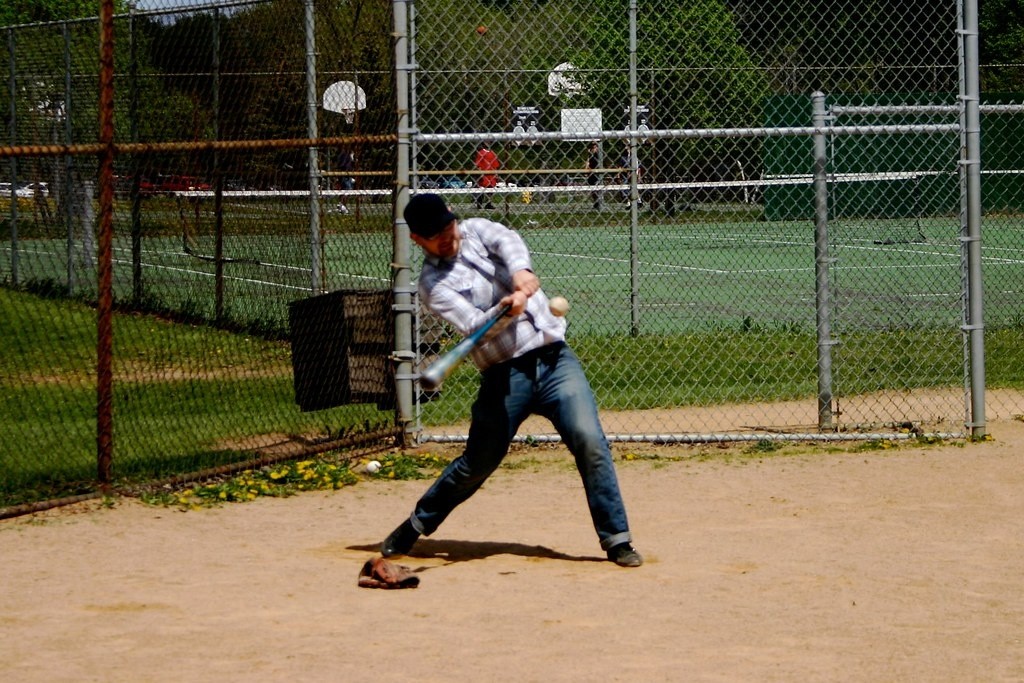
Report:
[288, 288, 445, 409]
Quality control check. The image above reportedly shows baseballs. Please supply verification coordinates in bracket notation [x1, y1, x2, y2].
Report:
[548, 296, 570, 317]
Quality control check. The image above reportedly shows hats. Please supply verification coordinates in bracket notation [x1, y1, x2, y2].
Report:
[403, 193, 459, 238]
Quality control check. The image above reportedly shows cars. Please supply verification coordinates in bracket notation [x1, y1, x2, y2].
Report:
[0, 180, 51, 201]
[415, 175, 516, 188]
[81, 168, 210, 201]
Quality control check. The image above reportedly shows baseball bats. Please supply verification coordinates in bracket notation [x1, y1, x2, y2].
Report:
[419, 303, 511, 390]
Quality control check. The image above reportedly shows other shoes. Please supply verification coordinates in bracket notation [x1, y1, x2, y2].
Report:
[625, 201, 631, 210]
[607, 542, 642, 566]
[382, 518, 420, 557]
[484, 203, 495, 209]
[477, 204, 481, 209]
[637, 201, 644, 210]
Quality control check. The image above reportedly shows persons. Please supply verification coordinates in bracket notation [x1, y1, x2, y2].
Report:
[586, 140, 600, 209]
[476, 139, 499, 210]
[619, 150, 643, 210]
[379, 193, 644, 568]
